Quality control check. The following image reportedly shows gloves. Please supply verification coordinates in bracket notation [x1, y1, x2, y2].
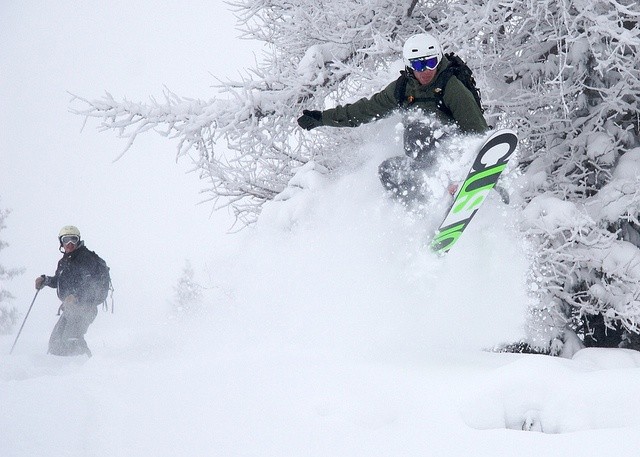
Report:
[297, 110, 323, 130]
[35, 274, 46, 289]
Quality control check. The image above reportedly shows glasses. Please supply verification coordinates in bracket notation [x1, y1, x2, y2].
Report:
[408, 58, 437, 71]
[61, 235, 79, 246]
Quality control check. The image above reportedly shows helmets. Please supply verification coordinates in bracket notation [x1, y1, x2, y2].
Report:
[403, 33, 442, 71]
[58, 226, 80, 243]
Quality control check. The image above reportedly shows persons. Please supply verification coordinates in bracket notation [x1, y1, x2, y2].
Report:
[35, 224, 98, 359]
[297, 32, 491, 248]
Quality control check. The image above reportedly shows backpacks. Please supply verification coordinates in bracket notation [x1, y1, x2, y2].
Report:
[395, 52, 484, 115]
[62, 252, 114, 314]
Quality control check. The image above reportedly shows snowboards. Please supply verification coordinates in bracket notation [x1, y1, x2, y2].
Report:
[429, 130, 519, 258]
